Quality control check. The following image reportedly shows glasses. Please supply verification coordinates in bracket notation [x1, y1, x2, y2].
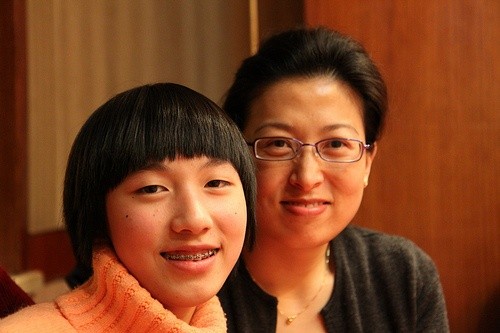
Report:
[246, 136, 371, 163]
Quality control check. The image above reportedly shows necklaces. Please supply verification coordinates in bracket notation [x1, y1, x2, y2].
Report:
[247, 243, 332, 324]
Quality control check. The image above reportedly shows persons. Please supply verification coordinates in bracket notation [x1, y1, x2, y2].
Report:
[0, 83, 259, 333]
[216, 25, 450, 333]
